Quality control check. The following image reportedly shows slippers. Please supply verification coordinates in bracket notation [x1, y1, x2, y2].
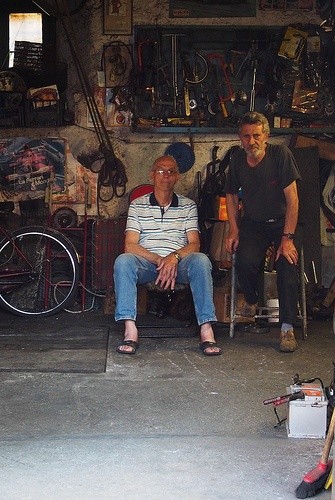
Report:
[199, 341, 222, 355]
[117, 339, 138, 354]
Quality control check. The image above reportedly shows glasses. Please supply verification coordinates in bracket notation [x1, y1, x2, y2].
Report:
[154, 168, 177, 176]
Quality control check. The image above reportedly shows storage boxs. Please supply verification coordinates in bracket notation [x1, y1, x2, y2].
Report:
[0, 41, 64, 130]
[105, 285, 147, 315]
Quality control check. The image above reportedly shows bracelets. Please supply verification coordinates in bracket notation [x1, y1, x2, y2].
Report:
[172, 252, 182, 261]
[281, 233, 296, 239]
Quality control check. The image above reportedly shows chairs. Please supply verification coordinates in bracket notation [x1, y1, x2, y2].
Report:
[90, 219, 126, 293]
[230, 223, 308, 340]
[128, 184, 200, 338]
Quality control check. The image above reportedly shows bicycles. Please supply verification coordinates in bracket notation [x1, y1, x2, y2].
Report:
[0, 209, 80, 319]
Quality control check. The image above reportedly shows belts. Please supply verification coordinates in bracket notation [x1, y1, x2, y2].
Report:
[244, 217, 280, 224]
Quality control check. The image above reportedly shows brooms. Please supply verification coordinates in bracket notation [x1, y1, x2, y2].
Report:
[294, 407, 335, 500]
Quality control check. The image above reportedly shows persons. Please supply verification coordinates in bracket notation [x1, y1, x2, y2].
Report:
[224, 113, 303, 353]
[112, 155, 222, 356]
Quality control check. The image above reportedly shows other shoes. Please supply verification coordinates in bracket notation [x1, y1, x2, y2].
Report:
[279, 330, 298, 352]
[241, 299, 258, 316]
